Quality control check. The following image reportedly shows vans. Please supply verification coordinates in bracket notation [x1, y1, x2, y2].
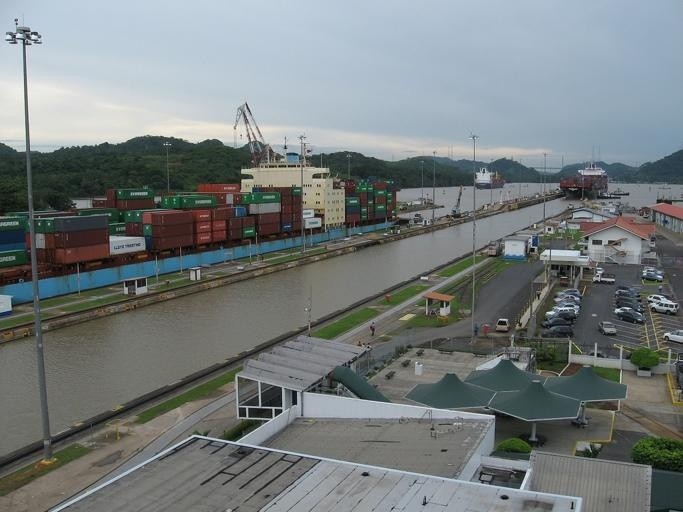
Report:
[647, 295, 680, 315]
[496, 319, 510, 332]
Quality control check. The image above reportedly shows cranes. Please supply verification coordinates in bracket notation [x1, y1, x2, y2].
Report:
[234, 102, 269, 167]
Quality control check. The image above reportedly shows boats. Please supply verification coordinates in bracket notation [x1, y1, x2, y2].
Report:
[477, 171, 506, 189]
[597, 188, 629, 198]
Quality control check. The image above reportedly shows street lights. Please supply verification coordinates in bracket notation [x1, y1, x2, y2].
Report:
[3, 26, 57, 464]
[347, 154, 352, 179]
[467, 131, 480, 344]
[542, 151, 547, 235]
[163, 141, 172, 193]
[297, 135, 309, 236]
[421, 151, 437, 224]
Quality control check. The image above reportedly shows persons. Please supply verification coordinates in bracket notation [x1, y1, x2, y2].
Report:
[365, 342, 371, 347]
[535, 287, 541, 300]
[357, 341, 361, 346]
[361, 343, 366, 347]
[369, 321, 376, 337]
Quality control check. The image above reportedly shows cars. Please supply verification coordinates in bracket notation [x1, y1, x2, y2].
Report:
[541, 288, 581, 339]
[642, 266, 666, 282]
[615, 285, 647, 324]
[599, 321, 617, 336]
[662, 330, 683, 344]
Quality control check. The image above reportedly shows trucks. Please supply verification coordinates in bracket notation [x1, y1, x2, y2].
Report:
[593, 268, 616, 285]
